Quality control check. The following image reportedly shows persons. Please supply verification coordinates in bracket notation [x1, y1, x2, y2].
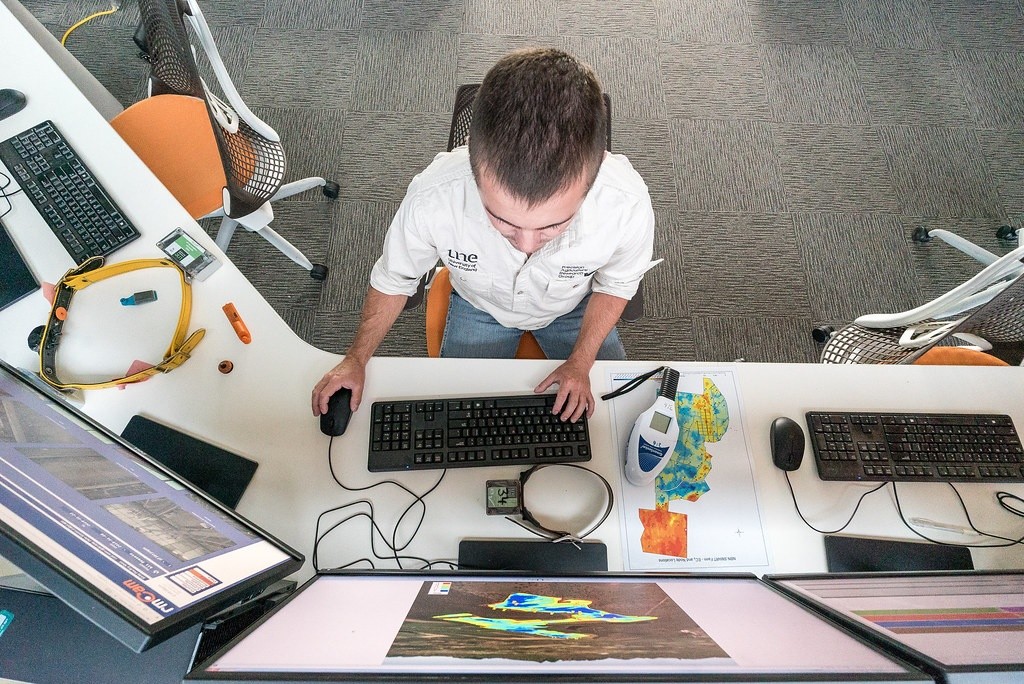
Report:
[311, 48, 655, 425]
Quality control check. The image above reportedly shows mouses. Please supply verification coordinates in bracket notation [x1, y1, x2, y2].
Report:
[770, 416, 805, 471]
[320, 387, 352, 436]
[0, 89, 27, 121]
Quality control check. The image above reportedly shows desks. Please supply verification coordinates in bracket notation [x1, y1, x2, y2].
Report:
[0, 0, 1024, 599]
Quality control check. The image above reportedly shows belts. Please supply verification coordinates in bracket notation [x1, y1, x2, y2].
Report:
[39, 255, 205, 389]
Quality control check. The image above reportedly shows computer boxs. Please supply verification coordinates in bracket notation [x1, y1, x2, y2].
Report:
[0, 574, 296, 684]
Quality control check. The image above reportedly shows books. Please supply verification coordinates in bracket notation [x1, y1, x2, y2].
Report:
[120, 414, 256, 511]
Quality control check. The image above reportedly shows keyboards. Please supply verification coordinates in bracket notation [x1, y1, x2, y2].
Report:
[0, 120, 141, 267]
[805, 411, 1024, 483]
[367, 394, 592, 472]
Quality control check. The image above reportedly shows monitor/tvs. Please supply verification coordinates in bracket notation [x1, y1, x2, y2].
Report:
[765, 537, 1024, 684]
[185, 537, 932, 682]
[2, 360, 305, 653]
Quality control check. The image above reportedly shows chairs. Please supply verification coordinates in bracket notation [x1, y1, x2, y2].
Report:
[403, 85, 644, 360]
[107, 0, 341, 282]
[811, 225, 1024, 366]
